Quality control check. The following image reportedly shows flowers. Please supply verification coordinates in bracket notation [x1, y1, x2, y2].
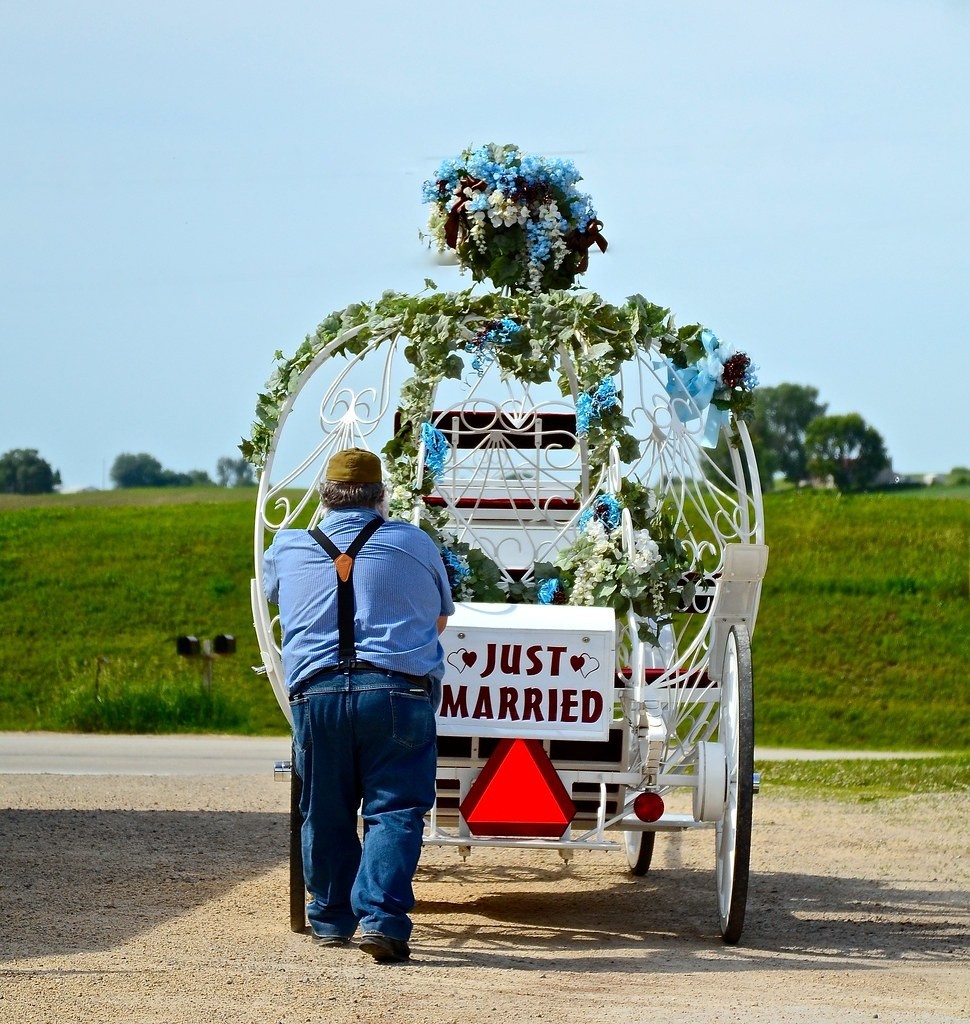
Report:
[239, 131, 758, 649]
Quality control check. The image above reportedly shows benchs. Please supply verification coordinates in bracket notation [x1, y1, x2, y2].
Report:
[392, 408, 618, 527]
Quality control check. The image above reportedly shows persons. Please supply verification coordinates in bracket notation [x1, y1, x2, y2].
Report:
[262, 445, 455, 963]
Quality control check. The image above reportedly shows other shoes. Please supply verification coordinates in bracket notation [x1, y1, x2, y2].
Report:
[310, 930, 346, 947]
[359, 931, 413, 964]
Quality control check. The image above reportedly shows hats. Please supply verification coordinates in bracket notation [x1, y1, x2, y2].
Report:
[326, 446, 385, 482]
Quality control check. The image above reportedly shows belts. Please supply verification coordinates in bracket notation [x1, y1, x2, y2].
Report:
[319, 663, 433, 690]
